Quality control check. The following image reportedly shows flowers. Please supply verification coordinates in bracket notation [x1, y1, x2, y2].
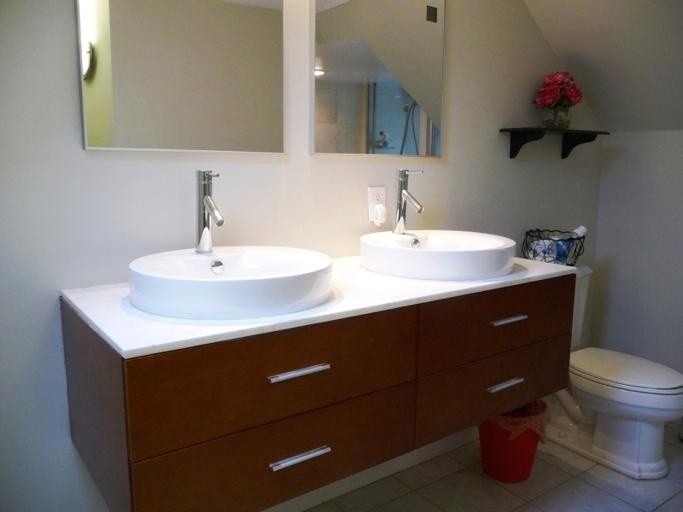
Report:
[534, 71, 583, 110]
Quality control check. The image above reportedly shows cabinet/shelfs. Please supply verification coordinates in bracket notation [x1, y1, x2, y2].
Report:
[59, 295, 416, 512]
[415, 274, 577, 449]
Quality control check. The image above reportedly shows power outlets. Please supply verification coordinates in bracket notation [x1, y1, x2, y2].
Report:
[368, 187, 386, 222]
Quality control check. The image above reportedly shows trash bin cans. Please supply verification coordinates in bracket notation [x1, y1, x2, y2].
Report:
[478, 399, 547, 483]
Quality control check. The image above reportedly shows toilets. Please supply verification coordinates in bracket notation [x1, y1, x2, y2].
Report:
[546, 263, 682, 480]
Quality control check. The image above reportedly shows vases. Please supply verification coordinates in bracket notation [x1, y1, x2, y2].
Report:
[549, 107, 571, 129]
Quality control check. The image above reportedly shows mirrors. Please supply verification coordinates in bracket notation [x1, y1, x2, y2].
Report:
[314, 0, 447, 157]
[76, 0, 284, 153]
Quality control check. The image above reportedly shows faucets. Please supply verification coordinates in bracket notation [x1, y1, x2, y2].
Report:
[195, 167, 225, 253]
[393, 165, 426, 235]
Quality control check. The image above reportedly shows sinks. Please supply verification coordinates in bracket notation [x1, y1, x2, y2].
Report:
[129, 246, 332, 312]
[354, 230, 514, 283]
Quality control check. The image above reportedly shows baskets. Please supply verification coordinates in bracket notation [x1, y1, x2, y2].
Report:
[522, 227, 585, 265]
